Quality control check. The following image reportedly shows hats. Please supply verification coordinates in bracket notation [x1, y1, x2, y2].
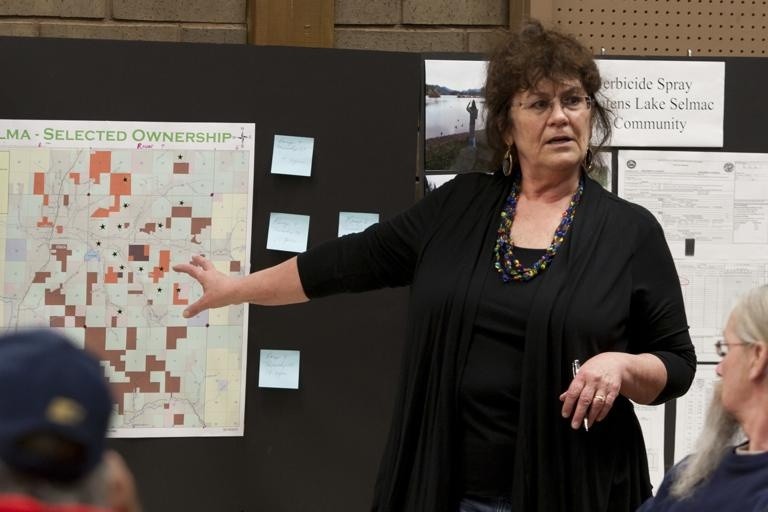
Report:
[1, 329, 115, 477]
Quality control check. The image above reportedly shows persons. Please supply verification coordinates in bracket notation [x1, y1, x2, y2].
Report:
[76, 443, 146, 509]
[0, 327, 121, 510]
[635, 281, 767, 512]
[170, 17, 698, 510]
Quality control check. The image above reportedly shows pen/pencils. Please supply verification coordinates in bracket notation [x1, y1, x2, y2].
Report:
[571, 359, 589, 432]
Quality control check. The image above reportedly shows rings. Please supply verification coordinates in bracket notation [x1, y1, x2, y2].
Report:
[593, 394, 607, 403]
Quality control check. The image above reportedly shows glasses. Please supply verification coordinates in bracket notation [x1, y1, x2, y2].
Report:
[510, 96, 595, 111]
[714, 340, 749, 356]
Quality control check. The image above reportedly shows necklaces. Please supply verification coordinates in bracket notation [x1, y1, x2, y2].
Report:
[492, 172, 586, 285]
